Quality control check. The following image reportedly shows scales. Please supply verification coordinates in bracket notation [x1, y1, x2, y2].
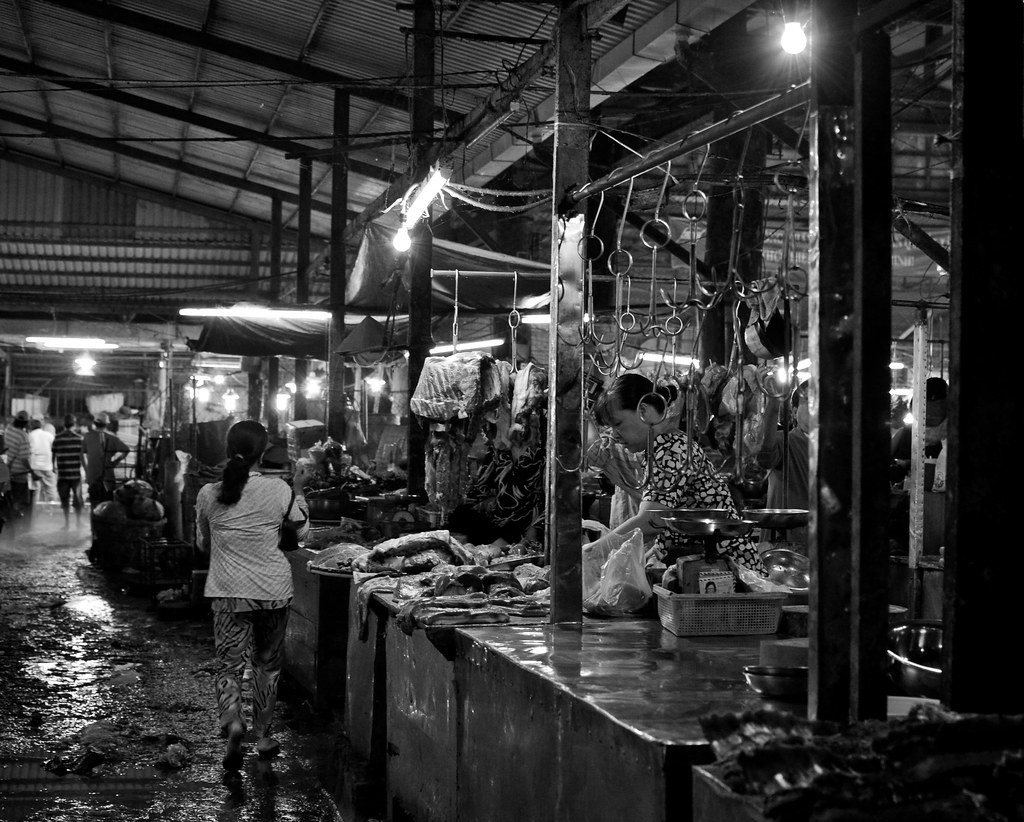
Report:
[369, 494, 424, 537]
[648, 507, 810, 593]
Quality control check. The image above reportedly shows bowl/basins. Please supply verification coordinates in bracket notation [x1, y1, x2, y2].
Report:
[759, 547, 809, 591]
[887, 625, 944, 690]
[307, 499, 347, 519]
[743, 665, 809, 701]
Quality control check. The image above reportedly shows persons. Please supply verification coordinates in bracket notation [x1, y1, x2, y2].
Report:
[582, 372, 768, 580]
[892, 377, 949, 481]
[195, 420, 310, 773]
[705, 582, 716, 593]
[27, 415, 58, 510]
[76, 412, 128, 508]
[51, 414, 87, 528]
[756, 365, 807, 550]
[4, 410, 30, 511]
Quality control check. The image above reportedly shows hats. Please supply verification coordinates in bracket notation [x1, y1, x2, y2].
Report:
[910, 378, 949, 426]
[94, 413, 110, 424]
[33, 413, 44, 419]
[790, 376, 808, 408]
[16, 411, 29, 421]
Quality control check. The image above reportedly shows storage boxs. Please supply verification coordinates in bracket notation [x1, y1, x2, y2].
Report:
[286, 419, 326, 461]
[652, 583, 787, 637]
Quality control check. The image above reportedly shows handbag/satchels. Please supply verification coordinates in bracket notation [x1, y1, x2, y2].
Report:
[279, 488, 308, 551]
[580, 519, 652, 615]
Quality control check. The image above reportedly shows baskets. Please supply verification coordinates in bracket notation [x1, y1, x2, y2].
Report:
[654, 583, 788, 636]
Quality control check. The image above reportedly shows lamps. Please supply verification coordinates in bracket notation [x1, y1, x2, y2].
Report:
[404, 337, 506, 359]
[195, 378, 213, 402]
[889, 347, 904, 369]
[76, 367, 95, 377]
[276, 365, 291, 410]
[406, 0, 455, 231]
[74, 352, 97, 367]
[221, 376, 239, 411]
[306, 361, 322, 386]
[183, 379, 195, 399]
[364, 369, 386, 391]
[284, 377, 297, 394]
[516, 313, 596, 324]
